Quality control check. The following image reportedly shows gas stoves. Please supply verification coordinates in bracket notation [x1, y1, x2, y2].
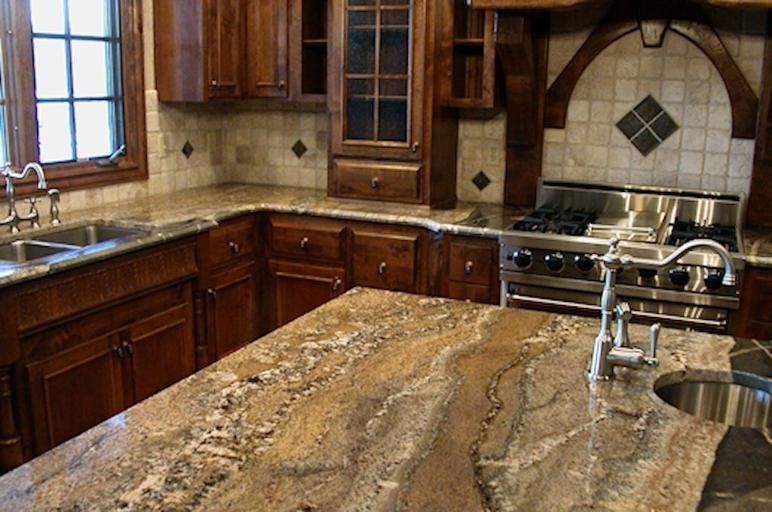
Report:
[498, 203, 746, 312]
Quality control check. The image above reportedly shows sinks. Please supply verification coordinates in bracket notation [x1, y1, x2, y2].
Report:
[1, 239, 83, 282]
[30, 221, 151, 248]
[655, 367, 772, 429]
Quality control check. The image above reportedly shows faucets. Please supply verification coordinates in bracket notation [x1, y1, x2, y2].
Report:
[1, 160, 47, 235]
[586, 236, 738, 379]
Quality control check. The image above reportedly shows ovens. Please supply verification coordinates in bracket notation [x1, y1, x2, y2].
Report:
[500, 282, 729, 335]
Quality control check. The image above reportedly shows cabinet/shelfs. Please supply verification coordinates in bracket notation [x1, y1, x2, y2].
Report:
[733, 266, 772, 341]
[1, 214, 501, 478]
[152, 0, 506, 210]
